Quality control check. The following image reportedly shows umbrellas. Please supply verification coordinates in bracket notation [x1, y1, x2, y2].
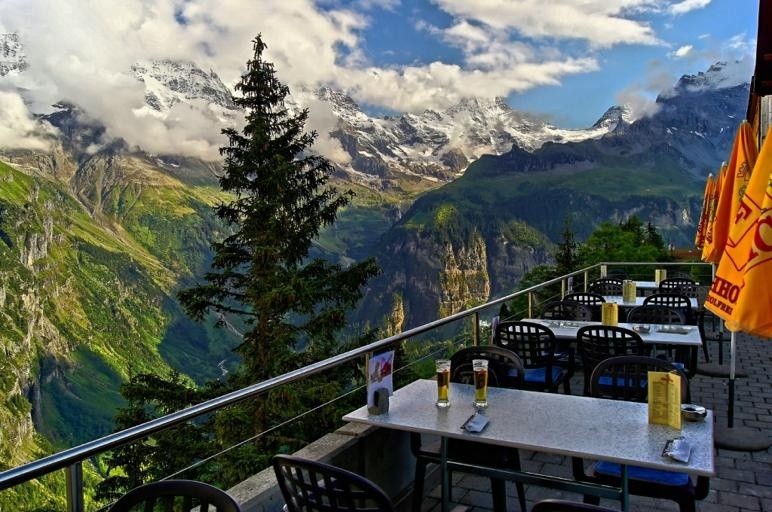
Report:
[695, 172, 718, 289]
[703, 122, 772, 340]
[701, 120, 758, 427]
[706, 160, 731, 363]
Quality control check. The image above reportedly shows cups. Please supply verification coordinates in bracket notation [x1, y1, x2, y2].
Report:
[472, 359, 489, 408]
[435, 359, 452, 408]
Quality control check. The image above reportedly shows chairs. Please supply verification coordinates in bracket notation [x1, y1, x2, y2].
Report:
[343, 279, 713, 512]
[105, 453, 401, 512]
